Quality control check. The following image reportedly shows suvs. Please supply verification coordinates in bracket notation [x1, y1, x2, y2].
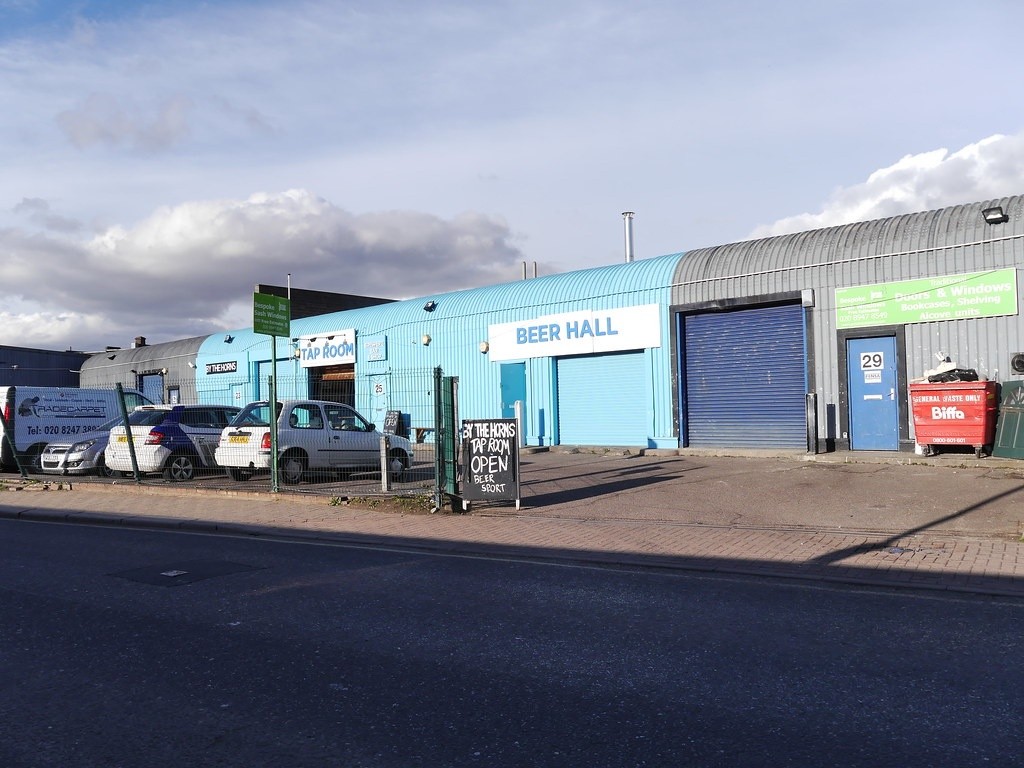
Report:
[213, 400, 414, 486]
[105, 404, 268, 484]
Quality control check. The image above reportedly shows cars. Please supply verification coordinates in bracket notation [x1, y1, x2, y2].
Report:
[40, 414, 129, 479]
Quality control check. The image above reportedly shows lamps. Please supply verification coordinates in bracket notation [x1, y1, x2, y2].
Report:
[424, 300, 436, 311]
[224, 335, 231, 343]
[982, 207, 1009, 225]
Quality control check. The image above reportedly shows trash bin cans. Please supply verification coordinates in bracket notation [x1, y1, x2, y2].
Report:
[908, 378, 999, 458]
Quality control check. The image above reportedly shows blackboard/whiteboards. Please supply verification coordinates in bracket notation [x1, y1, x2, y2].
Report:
[382, 410, 401, 436]
[460, 418, 520, 503]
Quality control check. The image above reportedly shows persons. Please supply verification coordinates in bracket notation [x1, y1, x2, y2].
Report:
[18, 396, 40, 418]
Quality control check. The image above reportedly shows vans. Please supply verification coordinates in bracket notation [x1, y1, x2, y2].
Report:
[0, 385, 155, 474]
